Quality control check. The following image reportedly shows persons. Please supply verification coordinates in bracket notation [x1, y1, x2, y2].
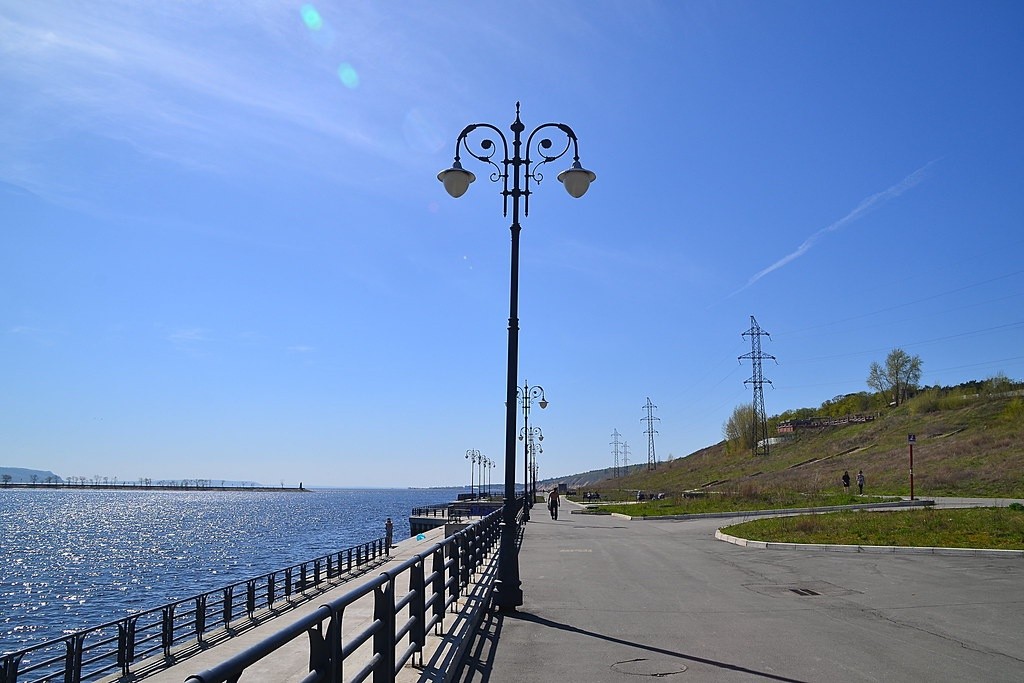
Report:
[647, 490, 655, 500]
[587, 491, 592, 502]
[842, 471, 850, 493]
[385, 517, 393, 547]
[548, 488, 560, 520]
[855, 471, 865, 497]
[594, 491, 601, 502]
[543, 489, 544, 493]
[637, 490, 644, 501]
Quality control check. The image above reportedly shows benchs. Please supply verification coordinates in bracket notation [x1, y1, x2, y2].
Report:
[583, 491, 601, 502]
[566, 492, 576, 496]
[636, 493, 654, 504]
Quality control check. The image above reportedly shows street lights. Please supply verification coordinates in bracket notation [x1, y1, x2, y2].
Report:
[504, 380, 549, 524]
[519, 425, 544, 509]
[435, 99, 597, 616]
[464, 447, 496, 501]
